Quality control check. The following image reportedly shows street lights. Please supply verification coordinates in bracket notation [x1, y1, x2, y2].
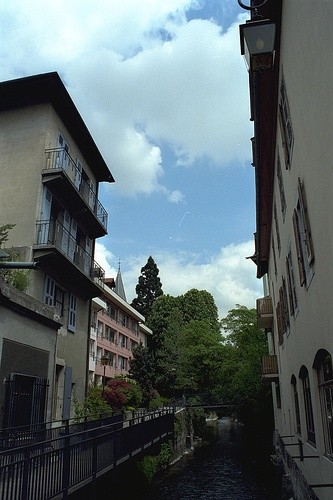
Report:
[99, 354, 110, 420]
